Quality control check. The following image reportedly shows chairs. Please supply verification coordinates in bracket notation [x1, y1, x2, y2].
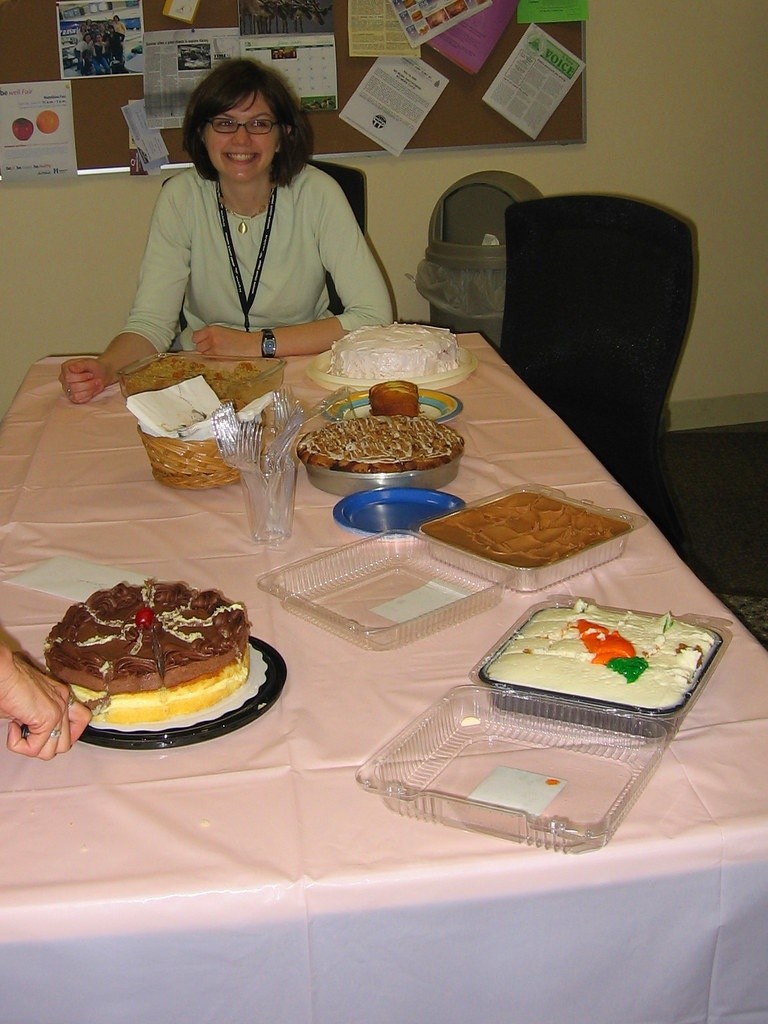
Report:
[495, 193, 698, 562]
[306, 160, 366, 317]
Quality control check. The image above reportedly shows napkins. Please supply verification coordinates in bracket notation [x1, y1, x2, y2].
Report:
[125, 374, 277, 443]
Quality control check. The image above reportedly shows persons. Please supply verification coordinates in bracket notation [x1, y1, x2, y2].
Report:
[0, 642, 92, 761]
[58, 58, 394, 405]
[73, 15, 129, 77]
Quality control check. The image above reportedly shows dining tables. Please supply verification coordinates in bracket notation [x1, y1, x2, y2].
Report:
[0, 327, 768, 1024]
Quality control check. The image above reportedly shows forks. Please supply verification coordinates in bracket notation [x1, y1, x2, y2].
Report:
[237, 421, 273, 540]
[212, 404, 241, 469]
[268, 390, 303, 541]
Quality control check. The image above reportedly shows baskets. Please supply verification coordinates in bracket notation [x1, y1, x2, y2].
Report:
[137, 399, 266, 490]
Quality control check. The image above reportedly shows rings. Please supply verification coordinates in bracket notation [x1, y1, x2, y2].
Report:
[67, 389, 71, 392]
[67, 698, 76, 709]
[50, 730, 61, 737]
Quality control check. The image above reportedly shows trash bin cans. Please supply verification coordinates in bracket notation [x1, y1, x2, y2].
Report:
[415, 170, 543, 356]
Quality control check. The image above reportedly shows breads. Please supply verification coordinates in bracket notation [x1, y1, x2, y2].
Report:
[299, 414, 464, 473]
[367, 380, 421, 417]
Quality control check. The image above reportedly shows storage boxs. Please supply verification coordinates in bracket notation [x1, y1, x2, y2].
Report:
[115, 351, 288, 403]
[353, 594, 737, 859]
[251, 480, 649, 649]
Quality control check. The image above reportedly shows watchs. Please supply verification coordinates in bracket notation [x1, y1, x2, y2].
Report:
[261, 328, 277, 358]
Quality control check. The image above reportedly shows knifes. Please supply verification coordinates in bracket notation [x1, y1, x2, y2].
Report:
[21, 695, 110, 738]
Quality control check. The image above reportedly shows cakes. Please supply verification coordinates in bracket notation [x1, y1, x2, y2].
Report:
[486, 599, 719, 708]
[42, 582, 251, 724]
[329, 322, 459, 379]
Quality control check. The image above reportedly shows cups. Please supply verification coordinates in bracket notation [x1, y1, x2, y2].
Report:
[235, 456, 306, 544]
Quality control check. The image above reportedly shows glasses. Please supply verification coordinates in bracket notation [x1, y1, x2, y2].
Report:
[204, 118, 279, 134]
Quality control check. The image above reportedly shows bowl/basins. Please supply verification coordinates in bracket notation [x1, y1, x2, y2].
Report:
[116, 351, 286, 427]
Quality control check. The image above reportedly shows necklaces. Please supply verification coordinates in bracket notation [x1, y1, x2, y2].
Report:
[218, 180, 275, 234]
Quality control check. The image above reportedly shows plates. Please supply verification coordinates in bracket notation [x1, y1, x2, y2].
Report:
[305, 348, 480, 392]
[321, 389, 464, 426]
[297, 426, 466, 497]
[332, 485, 467, 538]
